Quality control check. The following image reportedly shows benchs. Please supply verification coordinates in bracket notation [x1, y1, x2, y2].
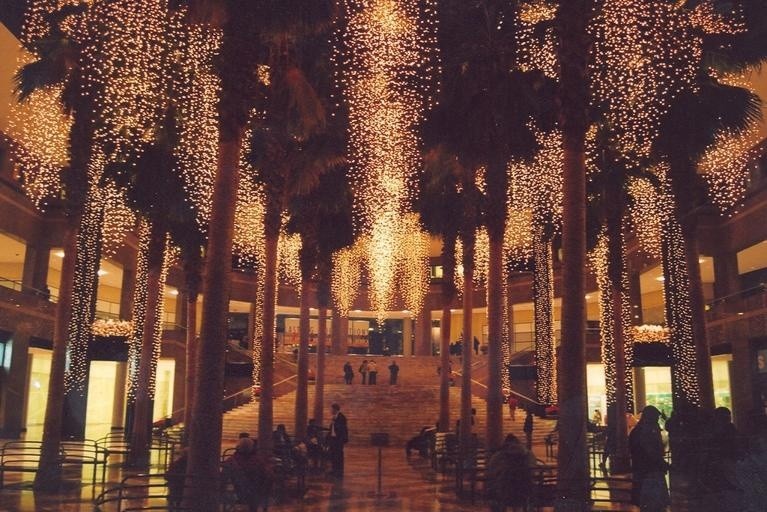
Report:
[0, 426, 328, 510]
[410, 425, 633, 511]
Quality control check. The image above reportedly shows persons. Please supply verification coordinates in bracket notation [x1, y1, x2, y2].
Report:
[42, 284, 50, 299]
[343, 360, 377, 385]
[625, 408, 644, 436]
[629, 404, 670, 511]
[470, 408, 476, 426]
[598, 445, 608, 467]
[508, 396, 516, 420]
[388, 360, 399, 384]
[592, 409, 601, 427]
[483, 433, 534, 511]
[327, 403, 347, 477]
[166, 446, 189, 511]
[714, 405, 736, 434]
[305, 418, 330, 433]
[219, 433, 257, 511]
[473, 336, 479, 355]
[273, 423, 289, 441]
[523, 409, 532, 450]
[228, 331, 249, 349]
[448, 340, 463, 356]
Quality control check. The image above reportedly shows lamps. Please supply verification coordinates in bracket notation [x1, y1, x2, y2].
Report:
[628, 321, 669, 348]
[91, 318, 135, 346]
